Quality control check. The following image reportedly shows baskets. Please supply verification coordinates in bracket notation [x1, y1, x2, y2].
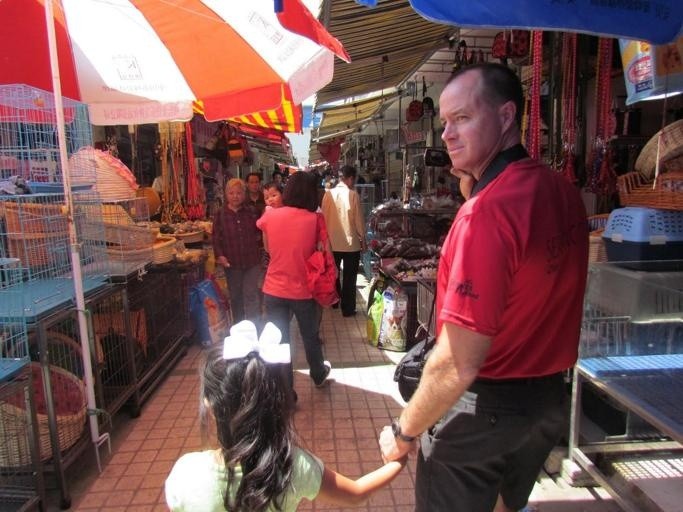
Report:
[1, 361, 88, 469]
[0, 147, 207, 266]
[601, 119, 683, 271]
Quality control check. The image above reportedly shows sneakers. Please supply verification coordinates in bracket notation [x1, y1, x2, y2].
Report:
[313, 360, 331, 388]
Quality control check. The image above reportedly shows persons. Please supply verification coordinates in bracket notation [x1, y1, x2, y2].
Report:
[374, 59, 591, 512]
[216, 163, 365, 390]
[164, 322, 409, 512]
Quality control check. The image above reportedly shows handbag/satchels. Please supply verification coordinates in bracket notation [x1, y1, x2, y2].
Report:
[366, 288, 409, 351]
[393, 336, 435, 401]
[309, 250, 340, 308]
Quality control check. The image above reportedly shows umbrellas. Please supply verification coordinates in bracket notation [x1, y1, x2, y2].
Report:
[0, 1, 352, 450]
[350, 0, 682, 46]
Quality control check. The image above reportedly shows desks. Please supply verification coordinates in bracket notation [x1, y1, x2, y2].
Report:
[571, 353, 683, 512]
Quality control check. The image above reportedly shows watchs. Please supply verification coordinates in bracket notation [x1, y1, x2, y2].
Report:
[390, 416, 416, 442]
